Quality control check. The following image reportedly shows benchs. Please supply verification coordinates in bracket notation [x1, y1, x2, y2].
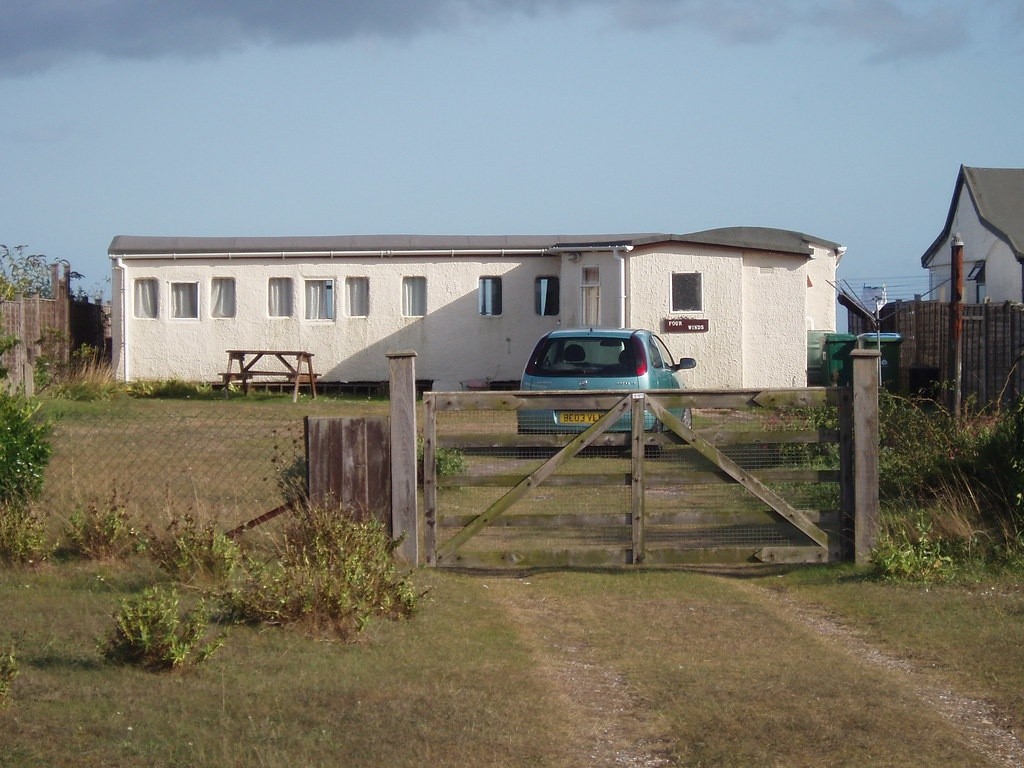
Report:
[219, 370, 324, 403]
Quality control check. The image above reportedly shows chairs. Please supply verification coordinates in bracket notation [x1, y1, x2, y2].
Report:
[564, 344, 585, 365]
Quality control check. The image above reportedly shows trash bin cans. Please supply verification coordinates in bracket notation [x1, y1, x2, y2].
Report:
[821, 332, 857, 386]
[857, 333, 904, 393]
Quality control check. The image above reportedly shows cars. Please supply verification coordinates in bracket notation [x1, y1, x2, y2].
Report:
[516, 326, 696, 453]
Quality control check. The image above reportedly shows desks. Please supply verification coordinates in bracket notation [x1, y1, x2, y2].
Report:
[226, 349, 317, 402]
